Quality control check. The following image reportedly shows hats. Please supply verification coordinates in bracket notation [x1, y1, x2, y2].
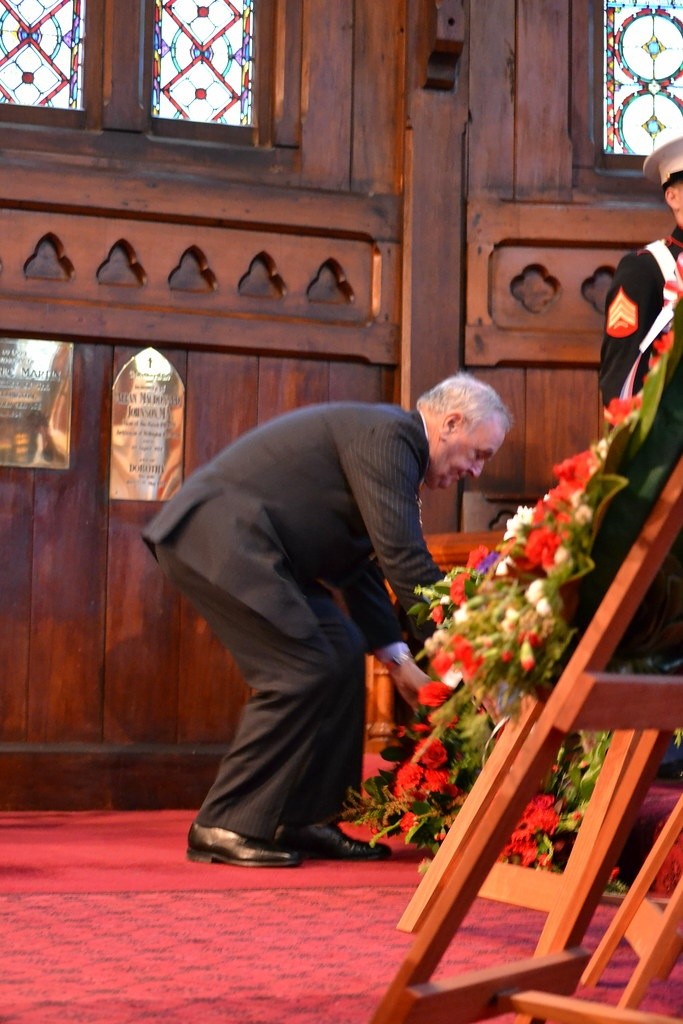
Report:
[642, 121, 683, 192]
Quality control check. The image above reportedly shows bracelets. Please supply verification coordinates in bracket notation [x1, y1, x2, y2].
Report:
[383, 651, 414, 672]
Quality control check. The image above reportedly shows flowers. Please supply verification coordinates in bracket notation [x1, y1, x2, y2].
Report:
[340, 322, 675, 894]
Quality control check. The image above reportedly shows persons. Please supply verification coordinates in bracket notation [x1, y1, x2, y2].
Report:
[599, 136, 683, 429]
[137, 372, 516, 868]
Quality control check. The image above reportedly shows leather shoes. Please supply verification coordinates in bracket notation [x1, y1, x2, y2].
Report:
[267, 821, 392, 860]
[187, 821, 304, 866]
